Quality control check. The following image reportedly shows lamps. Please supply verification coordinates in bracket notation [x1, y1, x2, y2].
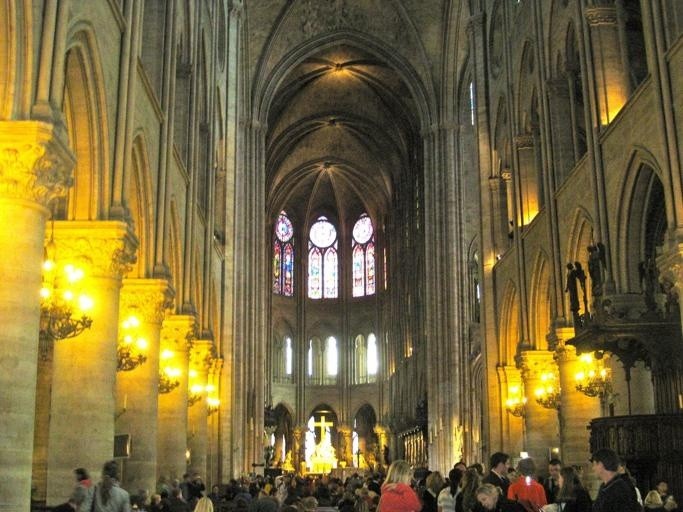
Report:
[535, 375, 559, 408]
[187, 370, 202, 407]
[38, 258, 93, 361]
[117, 316, 148, 372]
[575, 356, 611, 399]
[159, 349, 179, 393]
[207, 385, 220, 416]
[506, 387, 524, 417]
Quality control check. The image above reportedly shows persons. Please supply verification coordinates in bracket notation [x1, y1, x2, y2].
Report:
[59, 455, 682, 511]
[564, 262, 581, 312]
[587, 245, 603, 297]
